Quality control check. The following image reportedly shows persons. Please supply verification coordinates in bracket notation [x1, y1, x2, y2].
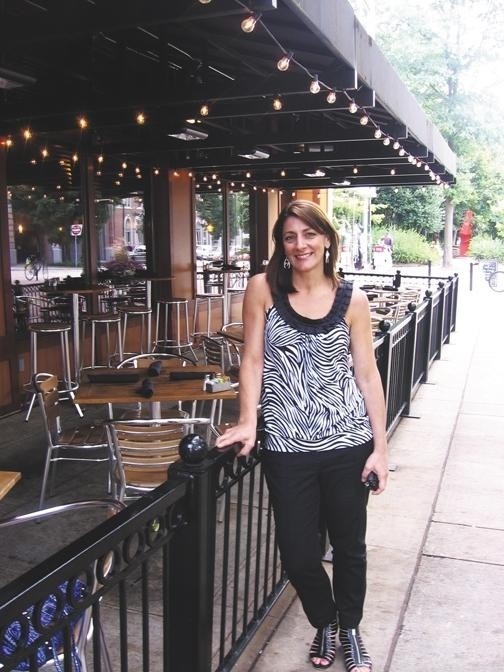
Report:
[214, 200, 388, 672]
[373, 237, 392, 274]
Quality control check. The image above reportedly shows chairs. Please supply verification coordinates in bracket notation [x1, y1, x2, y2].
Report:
[201, 262, 251, 291]
[12, 264, 148, 328]
[217, 322, 244, 368]
[1, 499, 127, 672]
[199, 335, 239, 425]
[351, 274, 419, 339]
[103, 417, 212, 580]
[31, 371, 119, 512]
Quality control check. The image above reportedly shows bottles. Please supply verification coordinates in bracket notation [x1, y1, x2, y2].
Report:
[203, 373, 224, 391]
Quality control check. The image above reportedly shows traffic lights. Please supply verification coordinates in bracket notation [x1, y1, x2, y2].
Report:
[207, 219, 216, 235]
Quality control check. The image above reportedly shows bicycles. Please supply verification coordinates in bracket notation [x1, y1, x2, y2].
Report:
[483, 261, 504, 292]
[23, 254, 48, 281]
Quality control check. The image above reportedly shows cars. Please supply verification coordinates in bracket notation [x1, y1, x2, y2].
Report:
[197, 245, 250, 269]
[132, 245, 146, 265]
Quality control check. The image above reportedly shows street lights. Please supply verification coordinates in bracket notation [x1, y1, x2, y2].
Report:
[121, 198, 125, 254]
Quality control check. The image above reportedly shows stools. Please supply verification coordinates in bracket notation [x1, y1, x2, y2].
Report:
[151, 298, 199, 363]
[192, 294, 225, 344]
[224, 287, 247, 327]
[80, 315, 124, 418]
[114, 307, 151, 365]
[24, 324, 85, 434]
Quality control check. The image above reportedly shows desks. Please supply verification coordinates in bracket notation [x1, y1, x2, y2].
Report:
[2, 470, 19, 497]
[72, 368, 239, 458]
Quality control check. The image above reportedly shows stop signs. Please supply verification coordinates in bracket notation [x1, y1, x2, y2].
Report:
[69, 224, 82, 236]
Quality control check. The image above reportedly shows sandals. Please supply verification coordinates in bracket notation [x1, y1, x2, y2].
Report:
[309, 618, 338, 668]
[339, 626, 373, 672]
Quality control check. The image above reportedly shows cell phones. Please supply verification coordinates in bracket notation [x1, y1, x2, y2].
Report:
[367, 473, 378, 492]
